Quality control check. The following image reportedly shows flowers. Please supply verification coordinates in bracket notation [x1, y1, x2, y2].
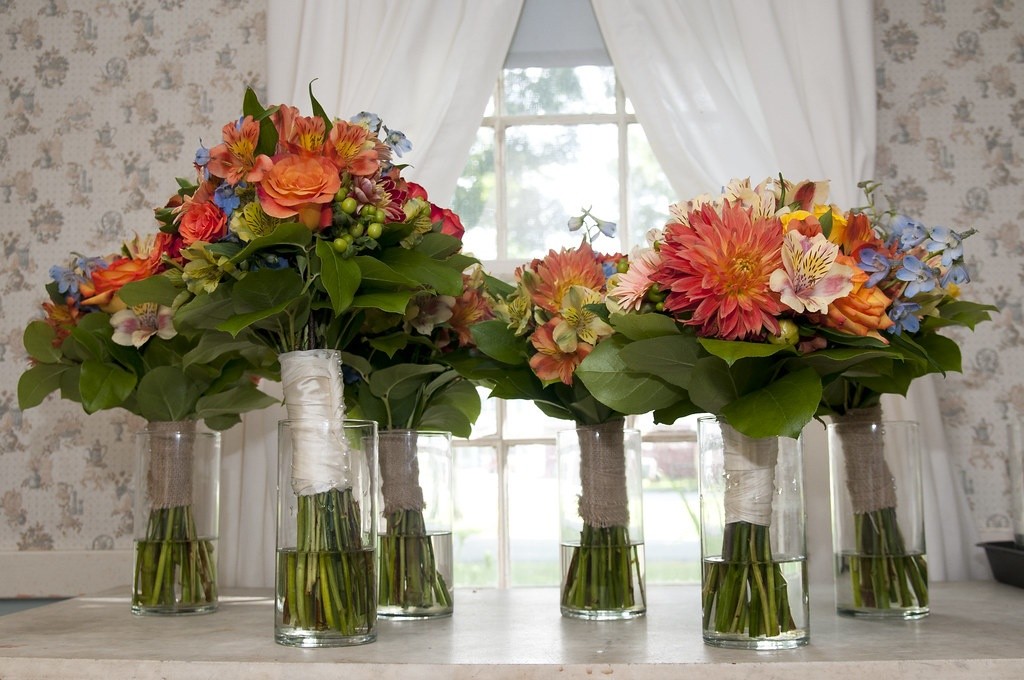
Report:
[340, 253, 499, 442]
[813, 180, 1001, 433]
[116, 79, 460, 353]
[576, 174, 912, 440]
[465, 206, 633, 423]
[17, 230, 278, 431]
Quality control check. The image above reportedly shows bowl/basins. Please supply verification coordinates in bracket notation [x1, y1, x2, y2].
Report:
[976, 541, 1024, 588]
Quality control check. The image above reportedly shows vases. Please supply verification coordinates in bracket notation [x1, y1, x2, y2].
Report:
[275, 419, 377, 651]
[357, 430, 456, 620]
[696, 418, 809, 649]
[131, 423, 221, 616]
[827, 421, 928, 622]
[555, 429, 648, 619]
